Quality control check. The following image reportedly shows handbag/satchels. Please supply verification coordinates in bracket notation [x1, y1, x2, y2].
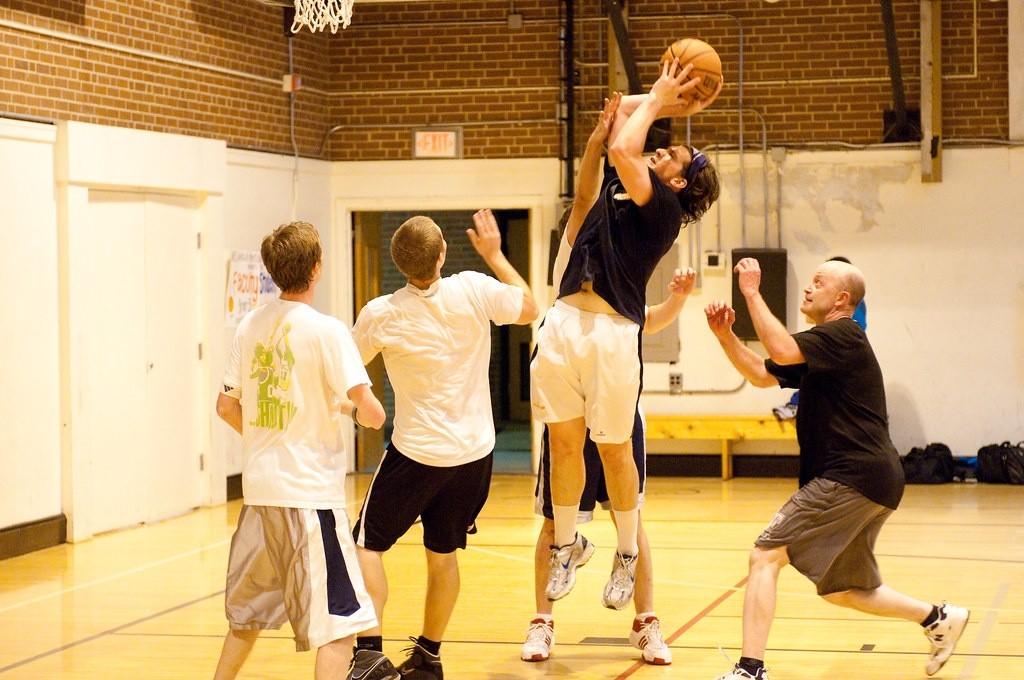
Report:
[976, 441, 1024, 484]
[900, 443, 966, 485]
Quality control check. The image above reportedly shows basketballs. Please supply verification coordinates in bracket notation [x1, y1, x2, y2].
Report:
[660, 38, 722, 103]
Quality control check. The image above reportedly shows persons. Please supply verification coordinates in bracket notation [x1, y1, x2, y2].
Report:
[516, 56, 723, 667]
[212, 222, 387, 680]
[344, 208, 541, 680]
[702, 251, 972, 680]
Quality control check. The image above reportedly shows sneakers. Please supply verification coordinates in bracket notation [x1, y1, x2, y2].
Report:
[602, 549, 638, 610]
[345, 646, 400, 680]
[715, 669, 766, 680]
[521, 618, 555, 661]
[628, 617, 671, 665]
[394, 635, 444, 680]
[925, 601, 970, 675]
[545, 532, 595, 601]
